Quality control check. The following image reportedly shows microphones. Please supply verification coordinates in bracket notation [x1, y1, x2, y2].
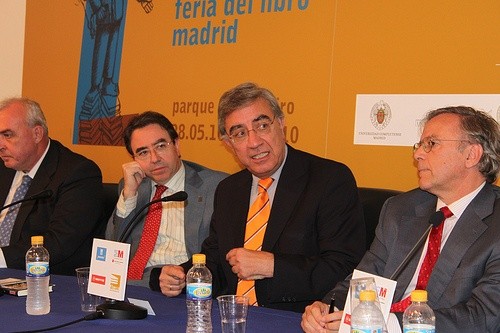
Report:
[0, 190, 52, 211]
[391, 210, 443, 279]
[96, 192, 188, 319]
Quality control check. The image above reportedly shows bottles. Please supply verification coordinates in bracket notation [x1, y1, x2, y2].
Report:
[185, 254, 212, 333]
[402, 290, 435, 333]
[350, 291, 388, 333]
[25, 236, 50, 315]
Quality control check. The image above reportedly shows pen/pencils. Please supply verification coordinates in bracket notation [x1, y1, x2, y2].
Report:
[329, 293, 335, 314]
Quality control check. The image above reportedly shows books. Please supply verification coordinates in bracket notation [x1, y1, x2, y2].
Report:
[0, 277, 53, 296]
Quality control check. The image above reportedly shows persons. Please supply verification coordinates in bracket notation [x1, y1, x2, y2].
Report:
[0, 97, 105, 277]
[148, 82, 367, 314]
[106, 111, 232, 290]
[301, 106, 500, 333]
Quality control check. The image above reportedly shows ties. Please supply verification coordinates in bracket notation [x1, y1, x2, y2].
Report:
[0, 175, 32, 248]
[236, 177, 274, 307]
[127, 185, 167, 281]
[390, 207, 453, 313]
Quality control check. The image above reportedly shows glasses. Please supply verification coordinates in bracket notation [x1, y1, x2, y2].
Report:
[133, 139, 175, 161]
[225, 112, 276, 142]
[414, 138, 479, 153]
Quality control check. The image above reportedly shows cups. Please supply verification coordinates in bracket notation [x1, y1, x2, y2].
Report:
[349, 277, 388, 333]
[76, 267, 101, 312]
[217, 295, 249, 333]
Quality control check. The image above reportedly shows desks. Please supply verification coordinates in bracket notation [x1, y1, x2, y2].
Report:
[0, 268, 306, 333]
